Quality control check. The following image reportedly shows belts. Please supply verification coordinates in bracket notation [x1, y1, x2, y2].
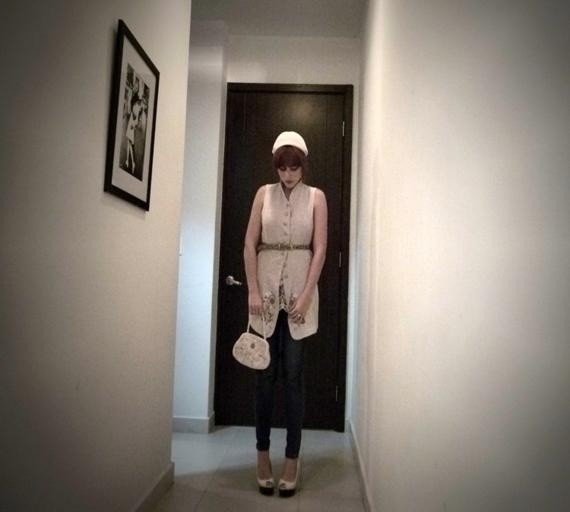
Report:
[253, 243, 312, 252]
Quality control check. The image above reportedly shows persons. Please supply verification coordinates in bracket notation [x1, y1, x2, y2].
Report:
[120, 81, 138, 174]
[240, 129, 331, 500]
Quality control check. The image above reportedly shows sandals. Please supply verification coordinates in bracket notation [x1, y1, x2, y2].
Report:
[278, 458, 300, 497]
[257, 457, 275, 496]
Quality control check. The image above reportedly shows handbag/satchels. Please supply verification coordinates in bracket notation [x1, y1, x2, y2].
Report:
[232, 332, 271, 370]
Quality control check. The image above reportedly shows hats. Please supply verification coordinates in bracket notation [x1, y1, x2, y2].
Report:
[272, 130, 308, 157]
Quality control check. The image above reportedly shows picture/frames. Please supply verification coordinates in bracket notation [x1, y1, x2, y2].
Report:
[103, 18, 160, 212]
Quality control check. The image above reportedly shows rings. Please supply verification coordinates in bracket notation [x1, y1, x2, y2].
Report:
[296, 313, 302, 320]
